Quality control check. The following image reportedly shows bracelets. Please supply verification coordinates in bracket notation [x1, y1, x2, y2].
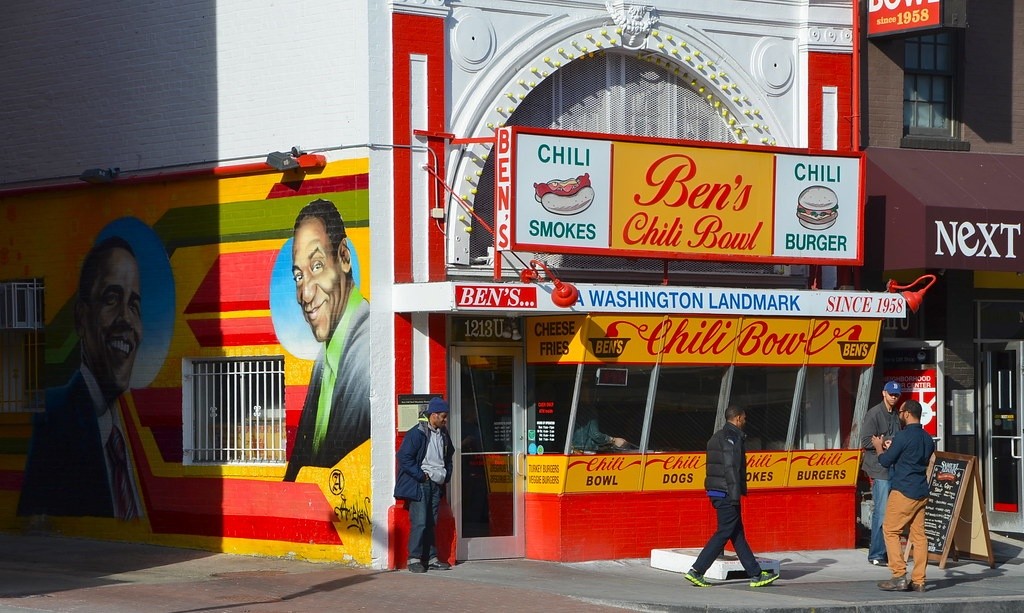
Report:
[612, 437, 615, 445]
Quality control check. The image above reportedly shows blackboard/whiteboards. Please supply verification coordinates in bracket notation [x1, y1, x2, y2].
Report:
[911, 452, 994, 562]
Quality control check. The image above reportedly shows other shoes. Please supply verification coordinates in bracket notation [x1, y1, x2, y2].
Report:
[426, 560, 449, 571]
[408, 561, 425, 574]
[869, 557, 886, 566]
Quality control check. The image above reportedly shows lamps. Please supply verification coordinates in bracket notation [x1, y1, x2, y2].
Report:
[78, 167, 118, 183]
[266, 146, 302, 170]
[887, 272, 938, 311]
[521, 259, 578, 308]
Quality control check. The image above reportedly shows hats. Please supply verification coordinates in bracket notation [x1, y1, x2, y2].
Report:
[884, 381, 902, 396]
[428, 397, 450, 414]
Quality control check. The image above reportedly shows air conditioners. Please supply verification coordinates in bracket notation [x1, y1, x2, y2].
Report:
[0, 284, 43, 331]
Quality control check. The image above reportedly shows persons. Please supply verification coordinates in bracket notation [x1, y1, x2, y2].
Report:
[861, 380, 902, 565]
[573, 381, 627, 450]
[871, 401, 934, 592]
[393, 396, 456, 573]
[684, 405, 779, 587]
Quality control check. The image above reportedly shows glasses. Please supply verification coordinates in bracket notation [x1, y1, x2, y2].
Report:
[897, 410, 910, 416]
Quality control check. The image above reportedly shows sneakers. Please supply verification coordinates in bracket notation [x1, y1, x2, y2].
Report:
[750, 571, 779, 588]
[685, 569, 712, 587]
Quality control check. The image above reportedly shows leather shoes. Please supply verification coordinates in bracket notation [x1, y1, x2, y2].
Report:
[877, 573, 907, 591]
[907, 580, 925, 593]
